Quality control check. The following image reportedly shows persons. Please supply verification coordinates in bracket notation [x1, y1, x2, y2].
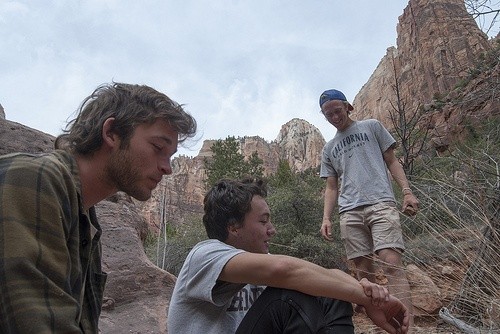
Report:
[319, 89, 420, 334]
[0, 81, 197, 334]
[167, 179, 410, 334]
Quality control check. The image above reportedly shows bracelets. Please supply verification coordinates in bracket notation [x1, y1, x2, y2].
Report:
[403, 193, 411, 196]
[401, 188, 412, 192]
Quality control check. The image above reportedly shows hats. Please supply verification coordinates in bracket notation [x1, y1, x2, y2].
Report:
[319, 89, 354, 111]
